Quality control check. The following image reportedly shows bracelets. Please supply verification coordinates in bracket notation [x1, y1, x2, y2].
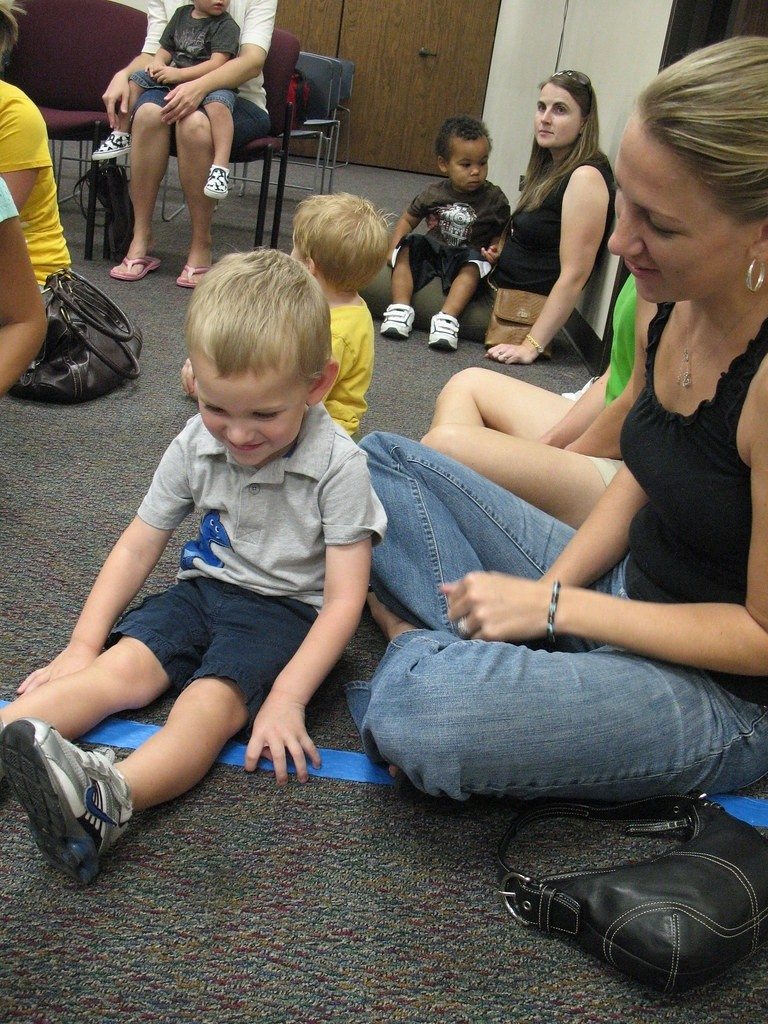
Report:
[527, 334, 544, 353]
[547, 580, 561, 648]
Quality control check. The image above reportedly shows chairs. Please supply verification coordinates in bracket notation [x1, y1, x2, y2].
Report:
[3, 0, 355, 248]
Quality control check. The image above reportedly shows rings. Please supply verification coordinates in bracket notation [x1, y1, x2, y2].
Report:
[504, 353, 509, 359]
[498, 350, 502, 355]
[458, 617, 470, 639]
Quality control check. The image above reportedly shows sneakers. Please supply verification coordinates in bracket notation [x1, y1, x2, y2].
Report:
[428, 311, 459, 350]
[92, 134, 131, 160]
[0, 717, 130, 887]
[380, 303, 415, 338]
[204, 167, 230, 199]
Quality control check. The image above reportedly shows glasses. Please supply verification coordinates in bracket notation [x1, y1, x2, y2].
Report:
[552, 70, 592, 99]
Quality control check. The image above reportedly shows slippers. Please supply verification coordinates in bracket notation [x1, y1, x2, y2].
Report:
[368, 574, 426, 628]
[110, 256, 161, 280]
[176, 264, 210, 287]
[395, 769, 427, 801]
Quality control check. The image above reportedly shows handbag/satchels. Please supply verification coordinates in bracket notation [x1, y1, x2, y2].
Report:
[286, 68, 309, 131]
[9, 269, 143, 404]
[485, 287, 553, 359]
[497, 796, 768, 996]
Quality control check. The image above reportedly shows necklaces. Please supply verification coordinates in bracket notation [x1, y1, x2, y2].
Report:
[674, 296, 764, 389]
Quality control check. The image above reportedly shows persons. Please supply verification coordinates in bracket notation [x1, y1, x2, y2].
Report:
[359, 74, 615, 363]
[0, 3, 69, 317]
[103, 0, 277, 286]
[182, 198, 391, 438]
[419, 274, 660, 532]
[91, 0, 241, 199]
[1, 248, 389, 886]
[0, 179, 49, 397]
[356, 37, 768, 806]
[380, 117, 512, 351]
